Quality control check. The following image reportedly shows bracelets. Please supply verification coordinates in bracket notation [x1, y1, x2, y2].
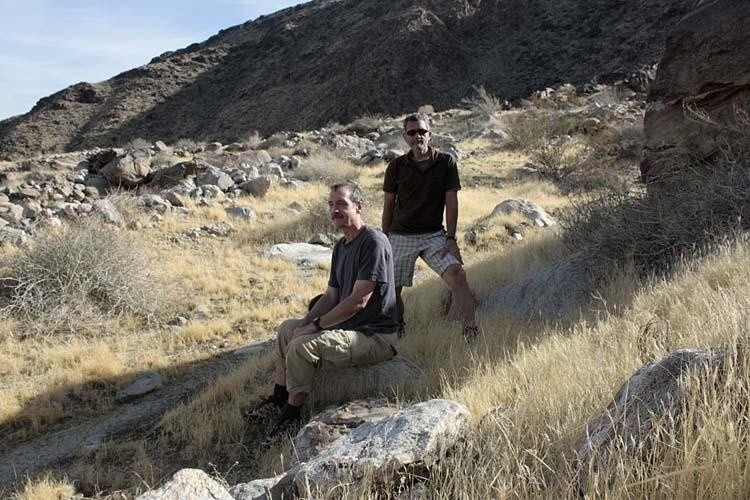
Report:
[446, 234, 457, 243]
[312, 317, 323, 331]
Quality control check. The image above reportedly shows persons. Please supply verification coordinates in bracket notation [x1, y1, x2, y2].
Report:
[380, 112, 481, 348]
[243, 181, 401, 452]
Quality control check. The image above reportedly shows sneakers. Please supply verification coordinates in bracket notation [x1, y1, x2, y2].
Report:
[260, 415, 298, 450]
[242, 394, 285, 424]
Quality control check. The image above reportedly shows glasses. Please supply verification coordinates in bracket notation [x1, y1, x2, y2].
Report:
[405, 128, 429, 137]
